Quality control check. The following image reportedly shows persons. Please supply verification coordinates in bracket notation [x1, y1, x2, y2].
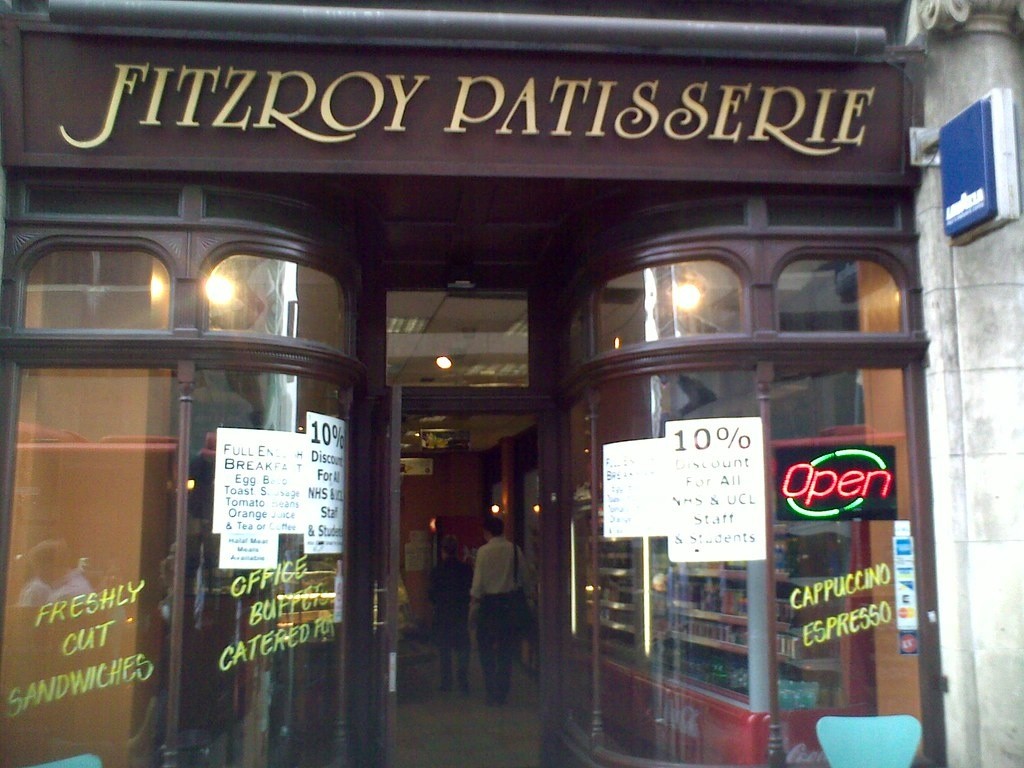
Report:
[16, 534, 96, 605]
[123, 536, 241, 768]
[468, 516, 534, 708]
[427, 533, 473, 696]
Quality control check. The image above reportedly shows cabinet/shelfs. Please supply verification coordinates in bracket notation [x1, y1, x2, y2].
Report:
[584, 521, 805, 667]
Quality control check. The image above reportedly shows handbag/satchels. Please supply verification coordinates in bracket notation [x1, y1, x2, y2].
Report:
[509, 542, 535, 642]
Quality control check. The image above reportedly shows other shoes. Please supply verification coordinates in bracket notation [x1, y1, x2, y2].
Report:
[483, 694, 497, 707]
[495, 691, 509, 705]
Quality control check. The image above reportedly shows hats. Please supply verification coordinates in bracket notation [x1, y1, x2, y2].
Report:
[454, 554, 476, 572]
[202, 433, 216, 458]
[441, 534, 459, 547]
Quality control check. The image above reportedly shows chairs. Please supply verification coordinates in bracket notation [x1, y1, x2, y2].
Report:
[815, 715, 924, 768]
